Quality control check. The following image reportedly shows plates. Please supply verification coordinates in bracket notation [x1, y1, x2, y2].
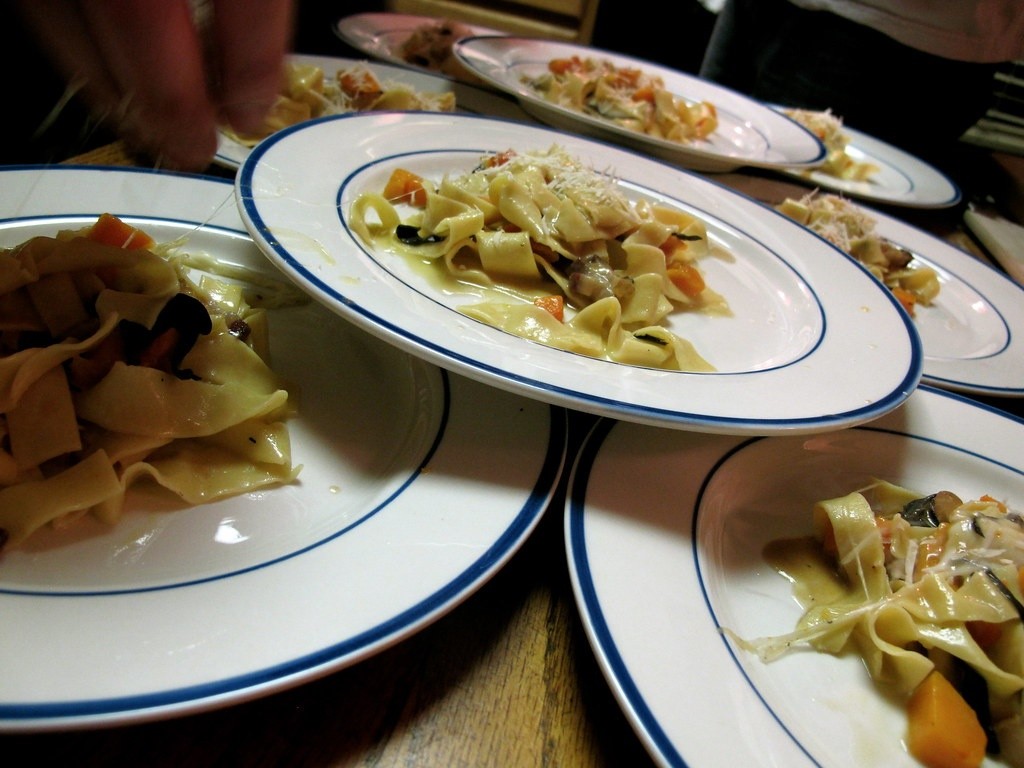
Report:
[452, 35, 825, 173]
[0, 167, 567, 731]
[212, 53, 557, 173]
[334, 13, 530, 98]
[687, 171, 1024, 395]
[562, 384, 1024, 768]
[234, 109, 923, 437]
[762, 103, 963, 209]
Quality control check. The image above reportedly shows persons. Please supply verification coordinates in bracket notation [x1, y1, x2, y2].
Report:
[0, 0, 399, 172]
[581, 0, 1024, 171]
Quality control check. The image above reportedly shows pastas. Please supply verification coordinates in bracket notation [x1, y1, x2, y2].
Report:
[775, 191, 942, 305]
[349, 141, 728, 377]
[523, 52, 718, 140]
[394, 17, 483, 86]
[0, 215, 301, 559]
[780, 106, 880, 185]
[793, 474, 1024, 708]
[215, 63, 456, 149]
